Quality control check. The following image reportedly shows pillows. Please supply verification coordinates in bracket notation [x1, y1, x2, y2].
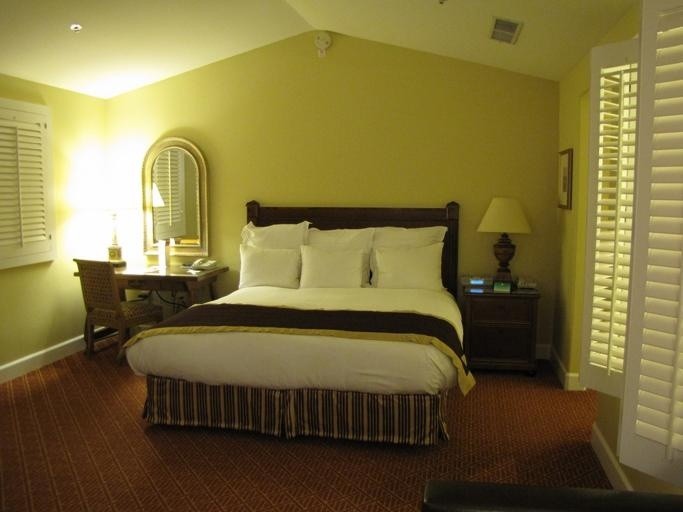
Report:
[373, 226, 447, 290]
[375, 243, 444, 291]
[242, 222, 309, 285]
[300, 246, 362, 287]
[307, 228, 373, 283]
[239, 247, 299, 288]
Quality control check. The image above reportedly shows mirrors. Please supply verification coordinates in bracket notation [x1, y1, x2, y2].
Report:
[144, 137, 207, 257]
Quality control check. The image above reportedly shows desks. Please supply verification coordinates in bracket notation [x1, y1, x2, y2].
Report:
[81, 263, 230, 337]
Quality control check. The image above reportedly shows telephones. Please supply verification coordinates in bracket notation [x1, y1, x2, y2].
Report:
[516, 277, 536, 289]
[191, 258, 216, 270]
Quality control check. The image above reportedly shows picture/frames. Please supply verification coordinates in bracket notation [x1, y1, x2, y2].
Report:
[557, 148, 574, 211]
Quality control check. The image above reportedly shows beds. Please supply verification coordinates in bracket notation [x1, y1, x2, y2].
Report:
[146, 201, 460, 444]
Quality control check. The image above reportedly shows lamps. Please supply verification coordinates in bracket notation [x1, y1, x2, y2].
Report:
[96, 185, 135, 267]
[152, 181, 164, 242]
[477, 197, 531, 287]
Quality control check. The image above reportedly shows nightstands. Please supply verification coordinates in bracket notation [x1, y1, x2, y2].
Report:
[463, 279, 539, 375]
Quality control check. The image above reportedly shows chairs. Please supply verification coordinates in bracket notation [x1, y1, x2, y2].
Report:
[75, 261, 152, 363]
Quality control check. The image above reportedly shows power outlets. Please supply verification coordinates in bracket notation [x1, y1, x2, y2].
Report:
[138, 289, 150, 305]
